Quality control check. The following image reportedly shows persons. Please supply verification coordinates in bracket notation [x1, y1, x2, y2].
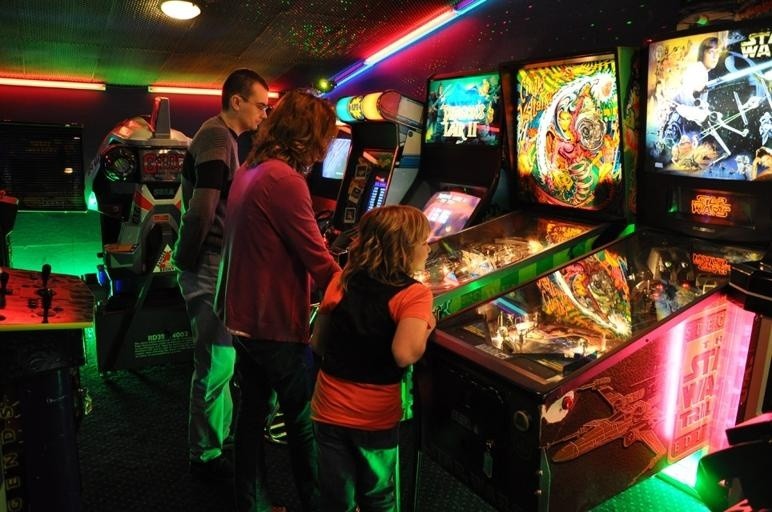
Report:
[171, 69, 270, 474]
[308, 204, 439, 511]
[659, 37, 719, 131]
[211, 86, 338, 512]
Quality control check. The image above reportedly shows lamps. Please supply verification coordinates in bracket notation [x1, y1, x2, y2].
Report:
[161, 1, 202, 21]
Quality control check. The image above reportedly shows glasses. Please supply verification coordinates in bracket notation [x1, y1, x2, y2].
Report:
[233, 93, 270, 111]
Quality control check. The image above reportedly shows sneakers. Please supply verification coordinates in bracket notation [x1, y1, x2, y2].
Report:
[186, 456, 234, 481]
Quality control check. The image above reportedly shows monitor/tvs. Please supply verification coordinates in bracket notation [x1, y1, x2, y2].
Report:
[319, 130, 351, 185]
[420, 183, 480, 241]
[355, 167, 390, 223]
